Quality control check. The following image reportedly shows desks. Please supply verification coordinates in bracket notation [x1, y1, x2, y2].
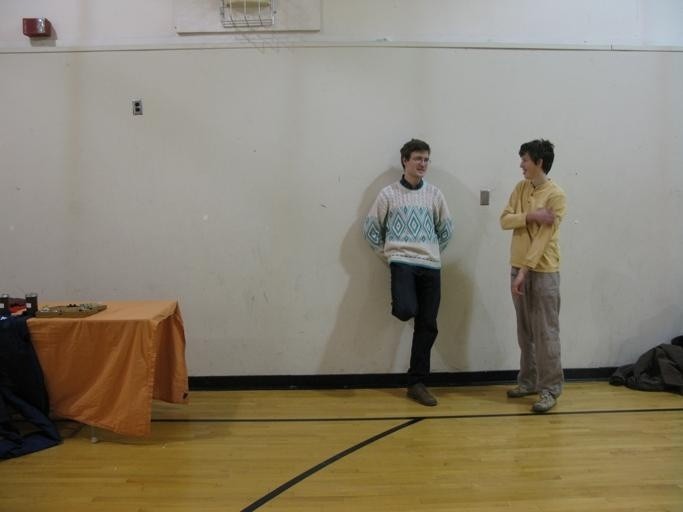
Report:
[11, 299, 190, 445]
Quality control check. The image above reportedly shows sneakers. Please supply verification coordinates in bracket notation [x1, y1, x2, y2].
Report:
[407, 384, 437, 406]
[508, 384, 538, 398]
[533, 392, 556, 412]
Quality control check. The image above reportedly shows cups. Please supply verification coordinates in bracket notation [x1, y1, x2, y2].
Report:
[24, 291, 38, 315]
[0, 294, 9, 317]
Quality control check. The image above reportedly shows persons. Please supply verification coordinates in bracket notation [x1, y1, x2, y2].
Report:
[360, 138, 456, 406]
[499, 139, 568, 414]
